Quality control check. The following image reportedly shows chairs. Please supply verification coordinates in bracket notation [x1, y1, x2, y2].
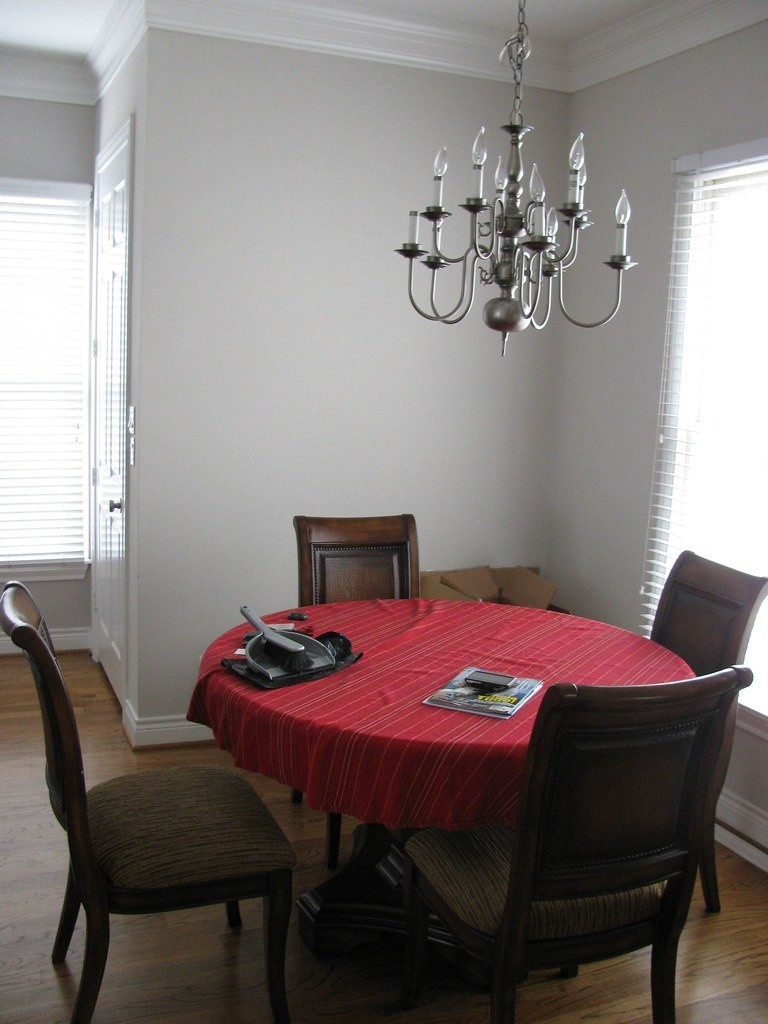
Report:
[401, 664, 754, 1024]
[0, 579, 299, 1024]
[289, 514, 419, 863]
[651, 550, 768, 913]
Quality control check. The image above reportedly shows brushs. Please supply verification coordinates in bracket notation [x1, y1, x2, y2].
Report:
[240, 606, 314, 673]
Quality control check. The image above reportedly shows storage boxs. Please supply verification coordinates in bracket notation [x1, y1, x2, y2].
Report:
[419, 565, 557, 610]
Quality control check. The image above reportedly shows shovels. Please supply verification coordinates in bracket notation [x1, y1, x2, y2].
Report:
[241, 605, 338, 682]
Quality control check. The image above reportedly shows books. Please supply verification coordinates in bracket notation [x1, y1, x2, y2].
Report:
[422, 667, 545, 721]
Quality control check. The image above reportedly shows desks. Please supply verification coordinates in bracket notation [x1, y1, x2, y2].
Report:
[184, 597, 698, 993]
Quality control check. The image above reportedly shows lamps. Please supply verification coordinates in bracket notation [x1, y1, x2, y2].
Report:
[394, 1, 638, 357]
